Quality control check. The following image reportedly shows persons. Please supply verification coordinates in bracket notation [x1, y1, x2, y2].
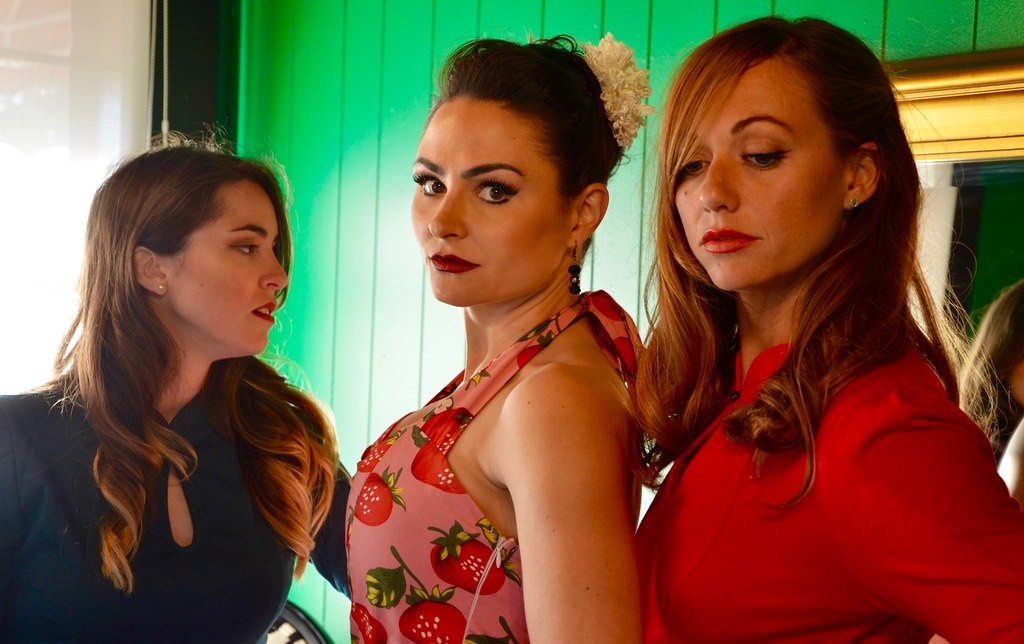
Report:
[344, 32, 655, 644]
[0, 129, 352, 644]
[627, 17, 1024, 644]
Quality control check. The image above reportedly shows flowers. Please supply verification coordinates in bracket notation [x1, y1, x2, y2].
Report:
[580, 30, 654, 149]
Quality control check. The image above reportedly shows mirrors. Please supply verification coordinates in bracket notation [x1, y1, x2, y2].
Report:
[880, 46, 1023, 502]
